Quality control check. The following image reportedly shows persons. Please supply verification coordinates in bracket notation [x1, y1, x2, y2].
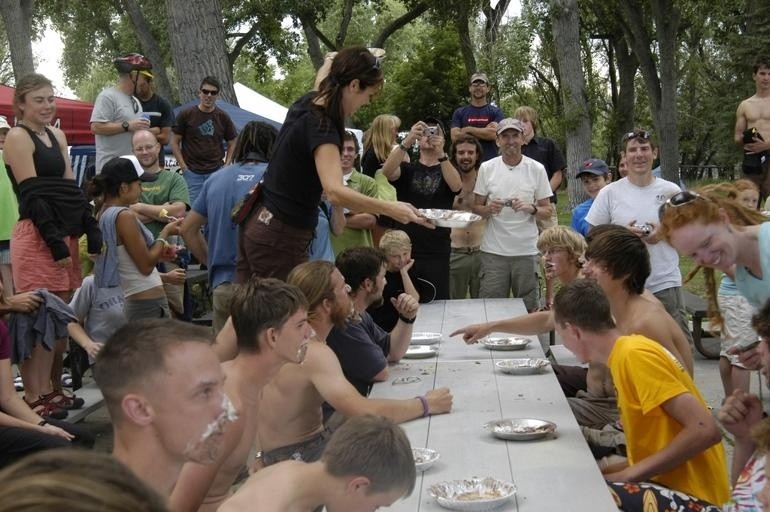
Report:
[1, 48, 768, 512]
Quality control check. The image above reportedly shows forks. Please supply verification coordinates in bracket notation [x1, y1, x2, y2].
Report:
[164, 215, 179, 221]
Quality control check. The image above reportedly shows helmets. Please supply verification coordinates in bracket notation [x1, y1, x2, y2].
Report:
[114, 53, 153, 73]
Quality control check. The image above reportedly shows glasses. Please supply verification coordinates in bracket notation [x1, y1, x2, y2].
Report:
[133, 141, 158, 153]
[372, 59, 381, 70]
[623, 131, 652, 142]
[343, 284, 352, 294]
[346, 131, 355, 137]
[472, 82, 486, 87]
[659, 191, 706, 223]
[201, 89, 219, 96]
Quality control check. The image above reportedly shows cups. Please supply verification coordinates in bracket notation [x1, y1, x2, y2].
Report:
[136, 112, 151, 130]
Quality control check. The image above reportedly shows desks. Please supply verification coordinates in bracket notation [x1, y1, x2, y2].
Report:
[315, 298, 618, 510]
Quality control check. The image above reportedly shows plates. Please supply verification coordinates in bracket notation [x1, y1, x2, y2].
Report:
[402, 332, 442, 359]
[496, 358, 551, 375]
[416, 208, 483, 229]
[412, 448, 440, 472]
[481, 337, 531, 351]
[485, 417, 558, 441]
[427, 478, 518, 512]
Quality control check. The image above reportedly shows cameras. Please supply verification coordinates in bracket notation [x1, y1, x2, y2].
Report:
[501, 198, 512, 207]
[736, 335, 761, 352]
[423, 126, 439, 137]
[633, 224, 651, 236]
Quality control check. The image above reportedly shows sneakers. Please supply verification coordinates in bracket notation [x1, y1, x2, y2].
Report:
[13, 376, 24, 391]
[61, 368, 73, 387]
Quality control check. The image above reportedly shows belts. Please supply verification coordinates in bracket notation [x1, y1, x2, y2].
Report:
[451, 247, 480, 254]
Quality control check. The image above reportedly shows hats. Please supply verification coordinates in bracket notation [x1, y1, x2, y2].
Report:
[130, 70, 153, 79]
[0, 117, 10, 129]
[471, 73, 489, 84]
[496, 118, 524, 135]
[576, 158, 609, 179]
[100, 155, 158, 184]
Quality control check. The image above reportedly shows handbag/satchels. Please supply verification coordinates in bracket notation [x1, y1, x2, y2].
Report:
[229, 183, 260, 229]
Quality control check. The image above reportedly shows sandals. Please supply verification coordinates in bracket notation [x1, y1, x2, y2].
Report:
[40, 391, 84, 410]
[22, 395, 68, 420]
